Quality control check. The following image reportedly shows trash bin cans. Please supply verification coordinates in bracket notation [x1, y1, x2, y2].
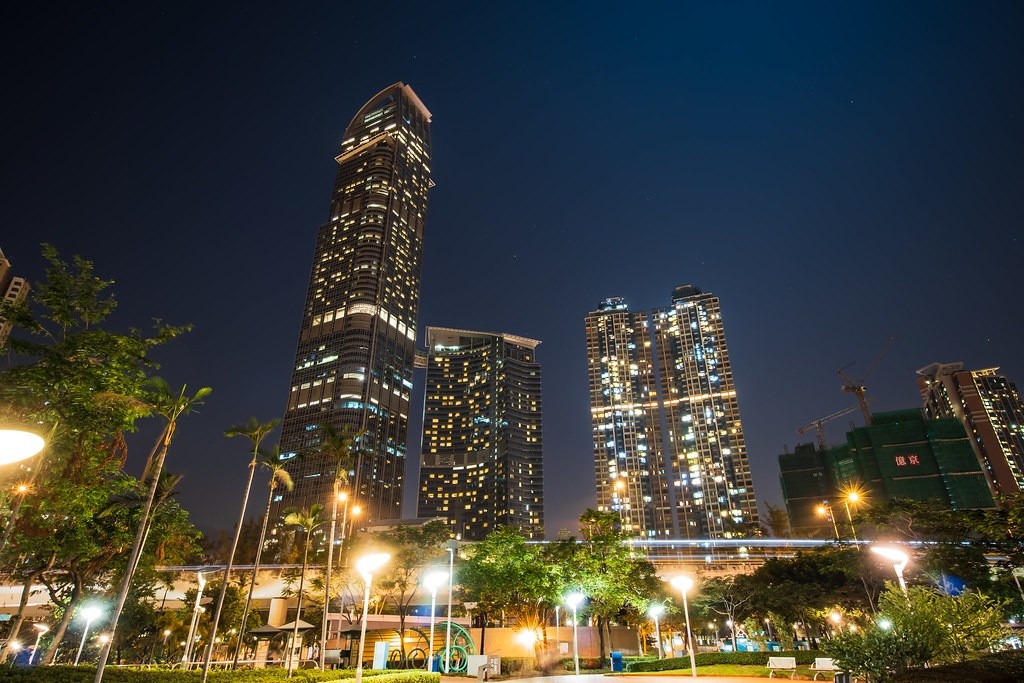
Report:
[609, 651, 624, 672]
[478, 664, 492, 681]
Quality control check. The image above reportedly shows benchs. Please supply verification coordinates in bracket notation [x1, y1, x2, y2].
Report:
[764, 657, 799, 681]
[808, 658, 840, 681]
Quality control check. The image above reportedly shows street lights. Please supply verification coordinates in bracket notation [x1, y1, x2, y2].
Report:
[831, 613, 844, 634]
[708, 623, 721, 651]
[725, 619, 737, 651]
[160, 630, 171, 658]
[338, 492, 349, 567]
[871, 544, 914, 615]
[355, 552, 391, 683]
[670, 575, 697, 677]
[616, 481, 625, 533]
[444, 548, 454, 673]
[649, 605, 665, 660]
[764, 618, 773, 640]
[8, 641, 22, 669]
[816, 505, 843, 550]
[555, 606, 561, 646]
[28, 623, 51, 665]
[565, 592, 584, 675]
[844, 489, 862, 551]
[73, 603, 105, 667]
[420, 566, 448, 673]
[344, 506, 363, 567]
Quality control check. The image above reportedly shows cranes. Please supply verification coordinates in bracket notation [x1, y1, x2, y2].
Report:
[794, 395, 876, 451]
[836, 361, 872, 426]
